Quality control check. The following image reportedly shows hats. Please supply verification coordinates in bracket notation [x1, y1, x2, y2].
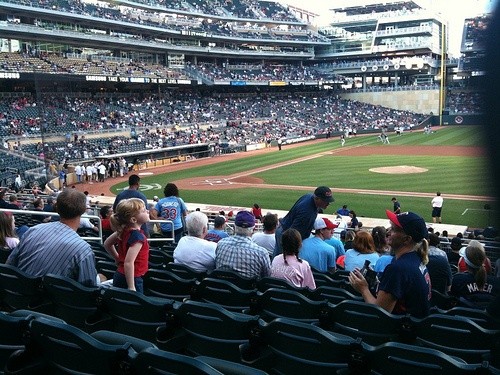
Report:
[235, 211, 255, 228]
[459, 247, 481, 268]
[314, 186, 335, 202]
[314, 218, 338, 230]
[215, 215, 228, 225]
[385, 209, 428, 243]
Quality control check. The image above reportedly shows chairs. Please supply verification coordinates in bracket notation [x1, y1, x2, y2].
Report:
[0, 192, 500, 375]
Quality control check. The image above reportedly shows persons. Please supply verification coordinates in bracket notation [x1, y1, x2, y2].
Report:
[431, 191, 444, 223]
[214, 211, 272, 280]
[335, 205, 350, 216]
[173, 211, 218, 272]
[4, 190, 107, 288]
[272, 228, 316, 289]
[0, 0, 500, 319]
[391, 198, 400, 215]
[152, 182, 189, 243]
[103, 198, 151, 295]
[347, 209, 432, 318]
[112, 174, 150, 246]
